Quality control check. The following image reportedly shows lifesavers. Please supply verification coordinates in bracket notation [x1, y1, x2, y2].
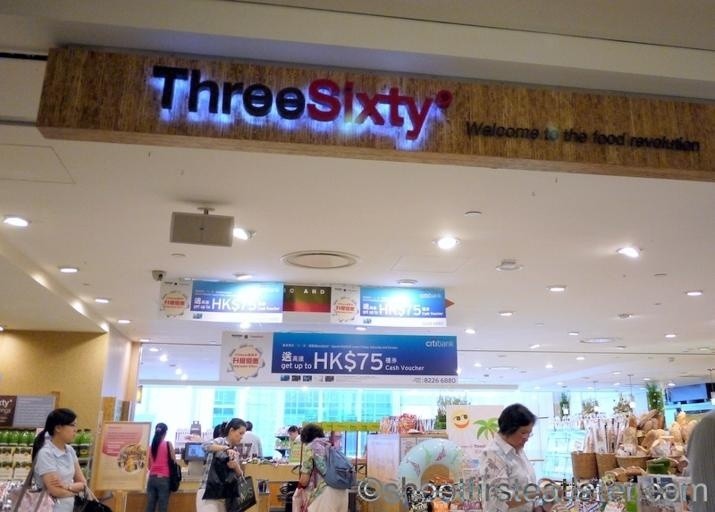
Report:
[396, 439, 474, 512]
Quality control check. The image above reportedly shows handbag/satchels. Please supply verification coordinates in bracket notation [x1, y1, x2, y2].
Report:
[0, 480, 56, 512]
[292, 487, 309, 512]
[167, 441, 181, 492]
[73, 484, 112, 512]
[202, 450, 256, 512]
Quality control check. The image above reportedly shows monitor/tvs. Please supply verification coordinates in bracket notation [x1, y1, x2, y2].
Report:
[665, 384, 711, 405]
[185, 442, 207, 460]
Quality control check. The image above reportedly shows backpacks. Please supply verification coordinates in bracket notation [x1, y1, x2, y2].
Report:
[315, 439, 354, 489]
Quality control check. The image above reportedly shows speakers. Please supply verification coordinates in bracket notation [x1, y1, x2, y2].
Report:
[169, 210, 235, 247]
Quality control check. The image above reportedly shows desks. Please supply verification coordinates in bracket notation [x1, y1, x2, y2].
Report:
[241, 457, 354, 511]
[99, 477, 201, 512]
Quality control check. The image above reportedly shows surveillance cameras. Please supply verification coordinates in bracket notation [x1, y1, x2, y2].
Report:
[151, 271, 166, 281]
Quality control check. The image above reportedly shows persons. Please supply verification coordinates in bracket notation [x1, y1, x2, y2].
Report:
[240, 421, 263, 458]
[196, 417, 246, 512]
[479, 403, 563, 512]
[30, 407, 87, 512]
[299, 423, 349, 512]
[146, 422, 176, 511]
[287, 425, 303, 445]
